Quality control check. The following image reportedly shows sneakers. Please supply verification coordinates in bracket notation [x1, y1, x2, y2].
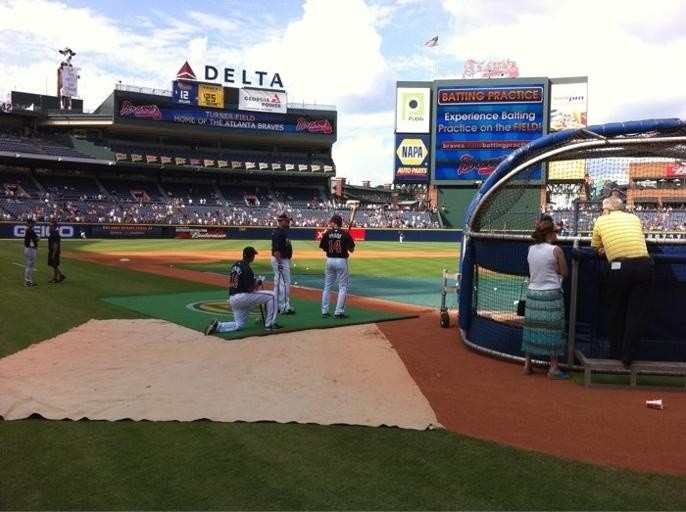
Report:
[335, 313, 349, 319]
[548, 370, 571, 380]
[523, 366, 532, 374]
[322, 312, 331, 318]
[24, 280, 38, 287]
[264, 322, 283, 330]
[205, 319, 218, 335]
[278, 308, 295, 315]
[49, 273, 65, 284]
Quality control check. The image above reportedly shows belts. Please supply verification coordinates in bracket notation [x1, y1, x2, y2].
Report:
[616, 257, 649, 263]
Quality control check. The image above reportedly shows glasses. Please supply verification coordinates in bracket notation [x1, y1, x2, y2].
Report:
[601, 207, 608, 211]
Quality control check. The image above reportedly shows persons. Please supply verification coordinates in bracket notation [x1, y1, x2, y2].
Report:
[590, 196, 656, 364]
[547, 196, 686, 240]
[46, 220, 66, 284]
[317, 214, 356, 319]
[23, 218, 41, 287]
[271, 214, 297, 315]
[400, 231, 404, 244]
[0, 169, 447, 232]
[520, 219, 571, 380]
[203, 246, 284, 336]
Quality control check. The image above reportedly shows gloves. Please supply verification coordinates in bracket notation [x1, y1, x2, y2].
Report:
[277, 262, 283, 271]
[258, 276, 265, 284]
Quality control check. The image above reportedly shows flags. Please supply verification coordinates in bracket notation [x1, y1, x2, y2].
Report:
[424, 35, 439, 48]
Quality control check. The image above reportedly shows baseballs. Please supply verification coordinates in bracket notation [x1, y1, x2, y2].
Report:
[255, 319, 262, 324]
[306, 268, 310, 270]
[295, 282, 298, 285]
[293, 263, 296, 267]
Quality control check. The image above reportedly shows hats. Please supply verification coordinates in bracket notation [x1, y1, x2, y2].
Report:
[278, 215, 292, 222]
[243, 247, 257, 257]
[533, 216, 562, 238]
[26, 218, 36, 224]
[330, 215, 342, 226]
[48, 217, 57, 226]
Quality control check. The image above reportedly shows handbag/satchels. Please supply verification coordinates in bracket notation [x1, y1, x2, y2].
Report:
[517, 300, 526, 316]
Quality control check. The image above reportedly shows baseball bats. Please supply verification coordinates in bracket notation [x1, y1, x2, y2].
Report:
[347, 207, 356, 233]
